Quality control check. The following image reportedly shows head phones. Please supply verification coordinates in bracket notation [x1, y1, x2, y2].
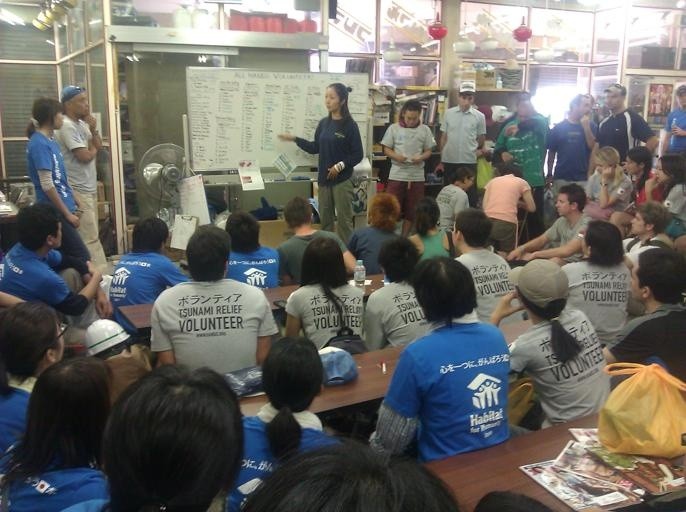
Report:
[609, 82, 626, 95]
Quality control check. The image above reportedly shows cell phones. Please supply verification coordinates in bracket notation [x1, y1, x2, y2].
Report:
[406, 160, 413, 163]
[273, 300, 286, 309]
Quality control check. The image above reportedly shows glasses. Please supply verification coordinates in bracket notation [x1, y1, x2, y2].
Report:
[54, 322, 67, 342]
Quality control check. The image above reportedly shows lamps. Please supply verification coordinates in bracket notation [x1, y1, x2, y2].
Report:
[451, 0, 479, 54]
[425, 0, 448, 43]
[480, 0, 501, 53]
[382, 0, 405, 69]
[536, 3, 560, 65]
[512, 1, 533, 47]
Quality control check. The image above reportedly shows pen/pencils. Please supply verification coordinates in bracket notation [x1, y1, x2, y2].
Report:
[380, 356, 387, 375]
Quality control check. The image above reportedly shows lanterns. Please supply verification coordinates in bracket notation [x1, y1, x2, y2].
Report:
[427, 15, 445, 41]
[512, 19, 533, 43]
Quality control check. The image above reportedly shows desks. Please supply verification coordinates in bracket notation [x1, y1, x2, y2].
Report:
[221, 320, 536, 424]
[422, 412, 685, 509]
[117, 272, 385, 332]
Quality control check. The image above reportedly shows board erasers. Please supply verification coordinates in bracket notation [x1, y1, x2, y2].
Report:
[311, 167, 318, 171]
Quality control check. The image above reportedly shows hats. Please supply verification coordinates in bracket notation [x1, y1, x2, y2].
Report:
[605, 83, 627, 97]
[509, 258, 570, 308]
[318, 346, 358, 386]
[459, 81, 477, 96]
[60, 85, 86, 105]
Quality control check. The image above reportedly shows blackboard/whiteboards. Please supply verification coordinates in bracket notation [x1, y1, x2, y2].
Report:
[185, 65, 370, 171]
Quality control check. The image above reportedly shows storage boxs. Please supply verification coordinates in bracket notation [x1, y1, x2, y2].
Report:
[93, 178, 105, 201]
[625, 41, 678, 74]
[96, 197, 112, 224]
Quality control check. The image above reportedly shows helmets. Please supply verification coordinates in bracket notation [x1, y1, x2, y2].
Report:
[84, 318, 130, 358]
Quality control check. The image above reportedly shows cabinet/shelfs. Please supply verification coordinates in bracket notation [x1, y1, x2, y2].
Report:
[364, 81, 444, 208]
[619, 64, 686, 147]
[447, 85, 532, 144]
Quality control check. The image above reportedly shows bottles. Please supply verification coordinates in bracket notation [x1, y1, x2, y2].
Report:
[354, 259, 365, 294]
[671, 119, 678, 135]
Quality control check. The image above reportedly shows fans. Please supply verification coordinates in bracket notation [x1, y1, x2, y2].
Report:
[135, 139, 194, 224]
